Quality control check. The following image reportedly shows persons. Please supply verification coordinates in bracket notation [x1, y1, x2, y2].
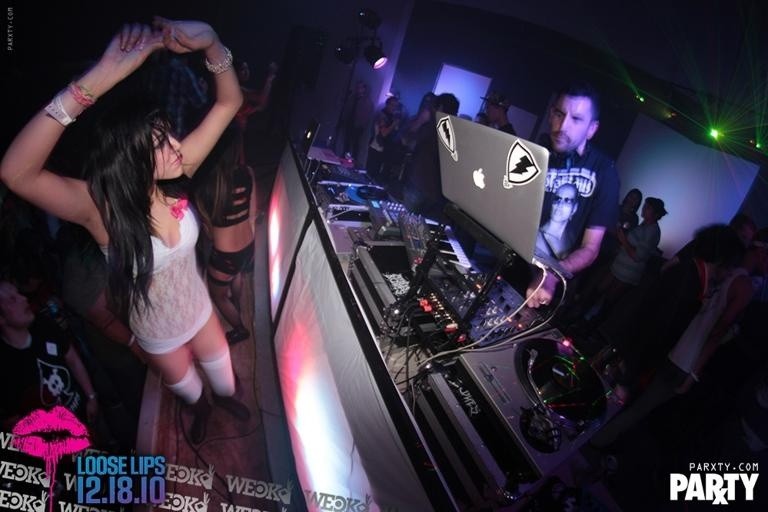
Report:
[366, 76, 765, 508]
[0, 0, 279, 512]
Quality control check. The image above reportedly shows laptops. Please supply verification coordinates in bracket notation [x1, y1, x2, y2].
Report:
[435, 111, 575, 280]
[304, 118, 343, 166]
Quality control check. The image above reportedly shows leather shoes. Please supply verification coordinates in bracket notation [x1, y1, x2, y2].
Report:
[227, 330, 249, 345]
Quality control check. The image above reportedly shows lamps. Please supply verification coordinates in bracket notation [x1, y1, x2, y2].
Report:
[332, 9, 389, 150]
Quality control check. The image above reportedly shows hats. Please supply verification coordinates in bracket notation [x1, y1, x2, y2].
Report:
[480, 91, 510, 111]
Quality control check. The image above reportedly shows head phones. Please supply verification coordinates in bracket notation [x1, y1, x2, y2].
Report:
[357, 186, 389, 200]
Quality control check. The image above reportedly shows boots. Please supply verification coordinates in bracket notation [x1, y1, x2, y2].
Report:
[181, 388, 211, 444]
[209, 391, 250, 421]
